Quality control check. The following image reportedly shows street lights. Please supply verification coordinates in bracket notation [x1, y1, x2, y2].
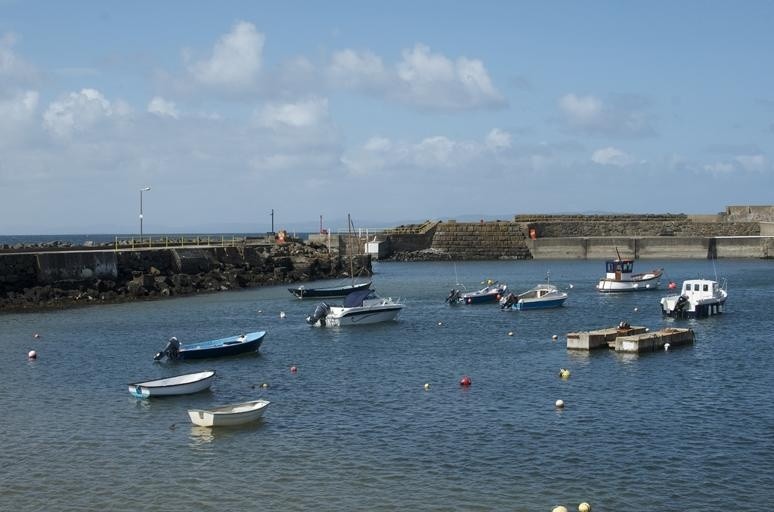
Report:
[139, 187, 150, 243]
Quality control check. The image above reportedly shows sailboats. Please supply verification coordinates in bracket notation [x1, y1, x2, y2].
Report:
[287, 213, 373, 300]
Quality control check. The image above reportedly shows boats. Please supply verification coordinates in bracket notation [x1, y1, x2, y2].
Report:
[153, 330, 267, 361]
[127, 370, 216, 399]
[498, 271, 569, 312]
[186, 399, 271, 428]
[445, 279, 508, 305]
[596, 247, 664, 293]
[304, 289, 407, 329]
[660, 253, 729, 323]
